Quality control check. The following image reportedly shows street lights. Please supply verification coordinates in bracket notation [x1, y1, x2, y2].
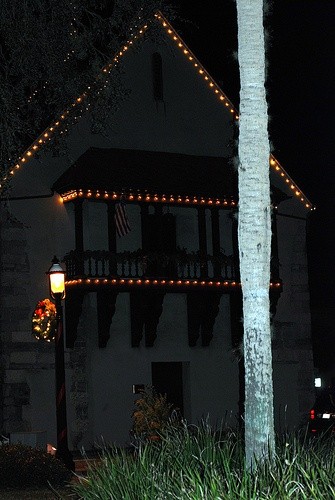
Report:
[47, 256, 77, 473]
